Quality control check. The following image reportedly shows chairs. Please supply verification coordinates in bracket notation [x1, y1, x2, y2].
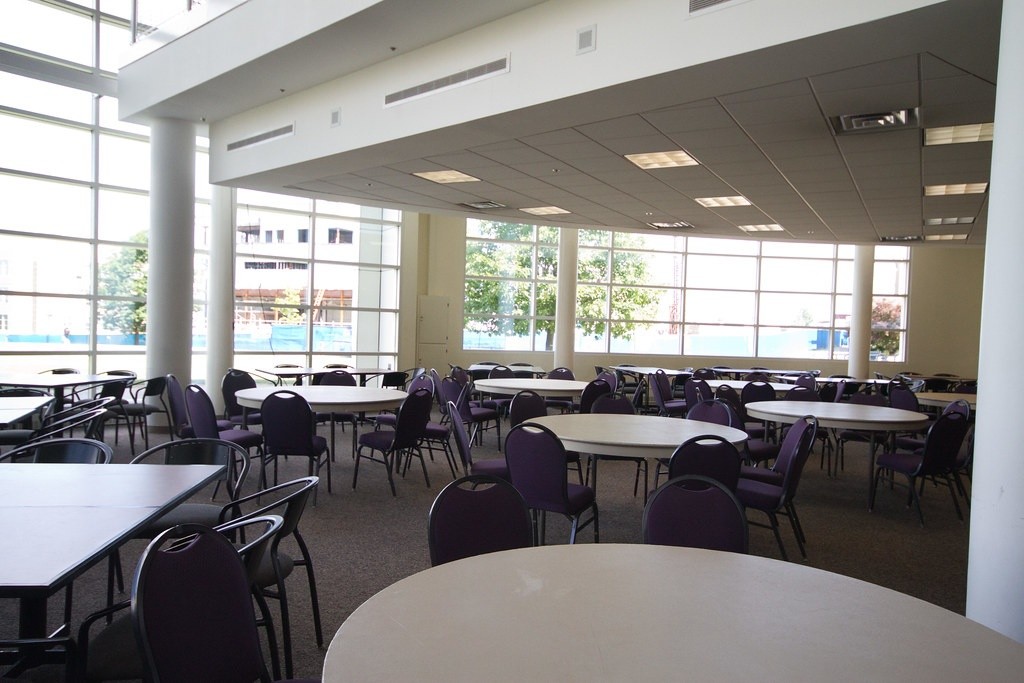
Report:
[0, 365, 977, 683]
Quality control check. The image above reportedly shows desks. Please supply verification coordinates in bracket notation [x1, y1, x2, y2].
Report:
[0, 395, 57, 431]
[0, 374, 136, 439]
[772, 375, 902, 409]
[709, 368, 818, 380]
[911, 392, 977, 425]
[0, 459, 228, 683]
[744, 400, 929, 508]
[466, 364, 547, 403]
[517, 413, 749, 512]
[609, 366, 692, 413]
[320, 543, 1024, 683]
[689, 376, 801, 405]
[237, 383, 409, 489]
[253, 366, 396, 386]
